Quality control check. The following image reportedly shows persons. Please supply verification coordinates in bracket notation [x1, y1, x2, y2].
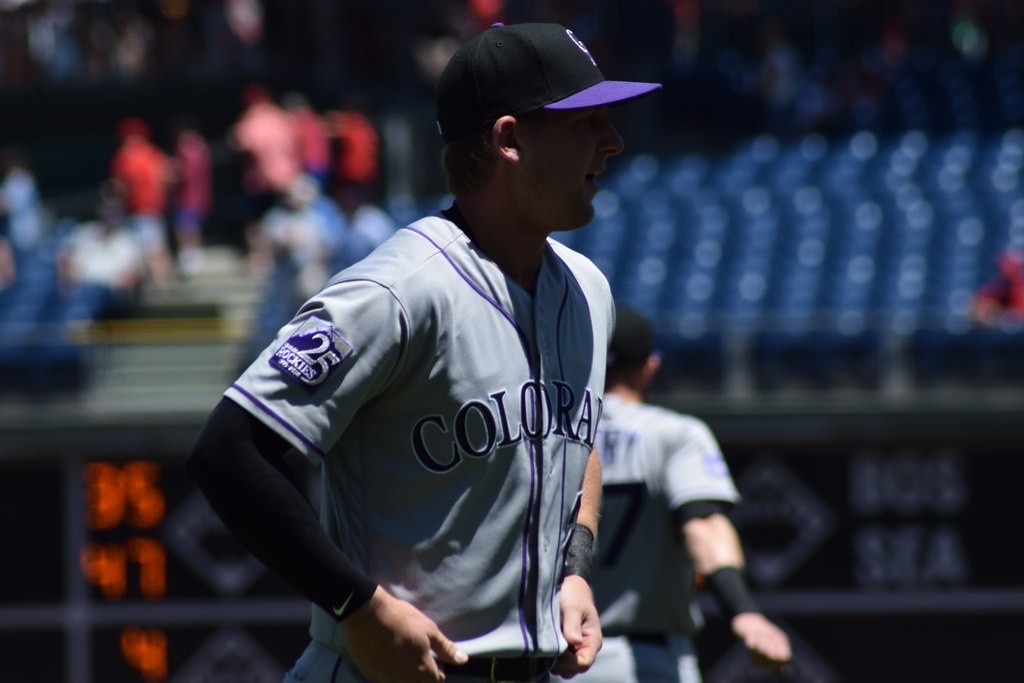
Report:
[184, 23, 662, 683]
[545, 310, 790, 683]
[0, 0, 1024, 378]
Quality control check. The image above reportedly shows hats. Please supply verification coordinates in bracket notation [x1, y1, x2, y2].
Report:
[437, 22, 662, 144]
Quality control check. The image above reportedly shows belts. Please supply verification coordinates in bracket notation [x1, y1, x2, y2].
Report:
[441, 657, 560, 680]
[628, 632, 667, 646]
